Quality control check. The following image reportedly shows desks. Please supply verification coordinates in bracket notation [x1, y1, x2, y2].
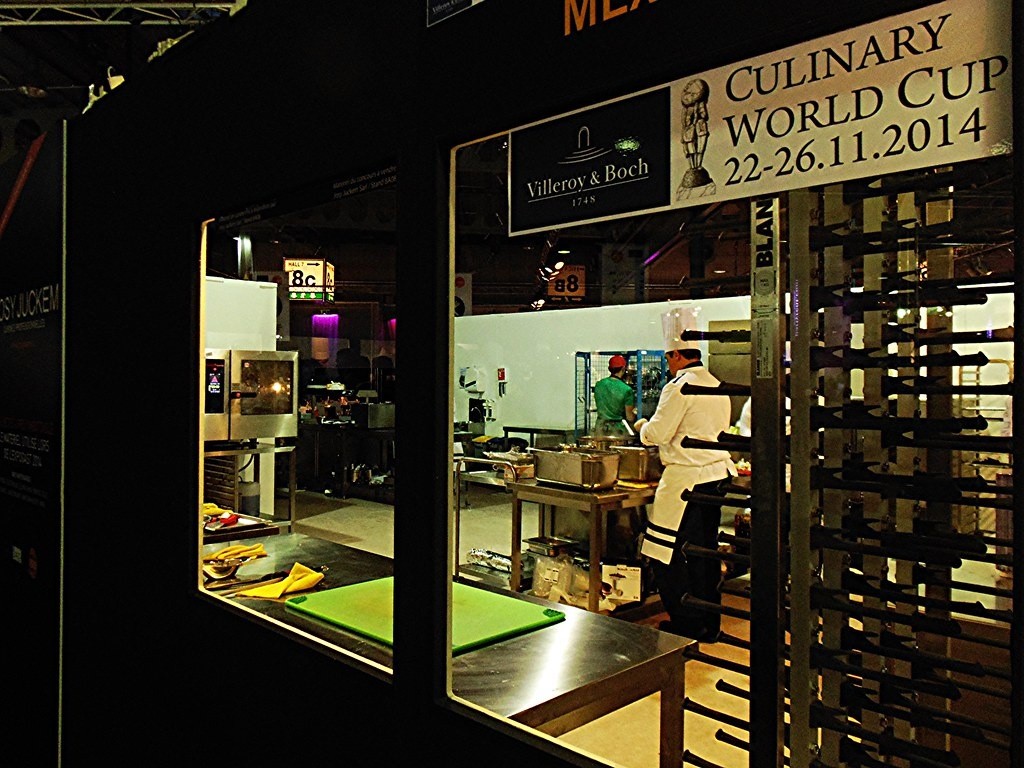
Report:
[502, 426, 581, 449]
[506, 479, 662, 620]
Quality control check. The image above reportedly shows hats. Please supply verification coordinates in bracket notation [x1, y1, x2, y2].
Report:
[661, 307, 701, 353]
[609, 356, 626, 368]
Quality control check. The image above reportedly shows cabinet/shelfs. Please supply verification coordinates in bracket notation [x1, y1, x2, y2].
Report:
[575, 345, 665, 441]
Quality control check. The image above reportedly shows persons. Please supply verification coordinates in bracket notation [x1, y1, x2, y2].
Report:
[593, 352, 635, 441]
[633, 326, 737, 646]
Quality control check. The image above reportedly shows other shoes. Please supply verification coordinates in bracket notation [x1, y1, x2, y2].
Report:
[694, 623, 720, 644]
[659, 621, 706, 639]
